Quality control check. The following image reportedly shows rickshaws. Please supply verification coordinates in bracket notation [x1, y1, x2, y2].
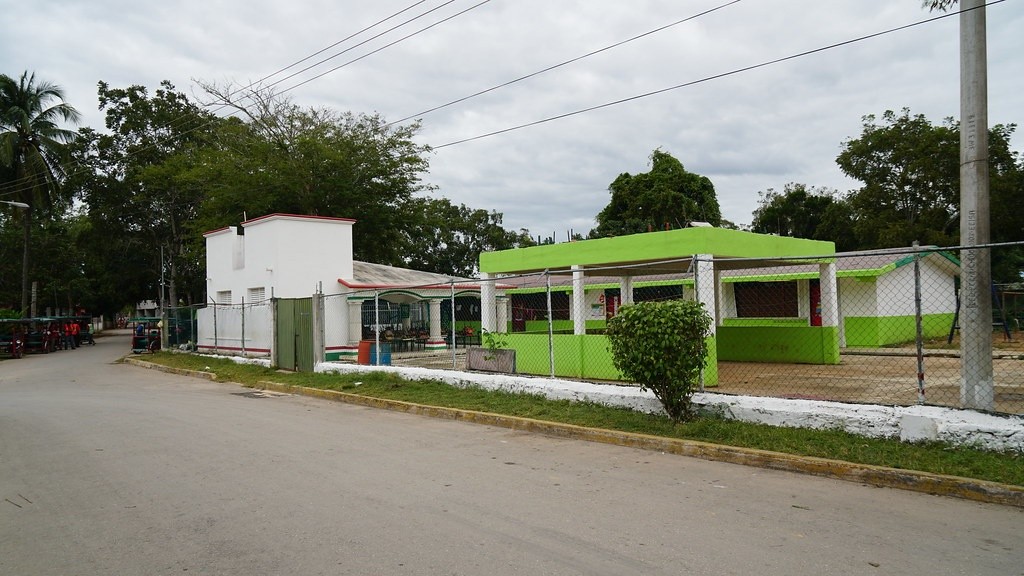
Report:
[0, 314, 91, 358]
[128, 317, 173, 355]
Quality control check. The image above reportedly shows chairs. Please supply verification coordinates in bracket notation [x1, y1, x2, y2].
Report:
[365, 328, 481, 351]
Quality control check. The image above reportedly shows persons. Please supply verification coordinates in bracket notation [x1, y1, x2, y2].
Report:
[86, 320, 96, 345]
[63, 319, 82, 350]
[136, 322, 146, 336]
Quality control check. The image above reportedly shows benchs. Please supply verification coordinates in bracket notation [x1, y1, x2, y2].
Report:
[0, 323, 164, 349]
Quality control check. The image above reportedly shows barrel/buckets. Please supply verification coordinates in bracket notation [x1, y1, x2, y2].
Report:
[369, 343, 391, 366]
[358, 340, 382, 364]
